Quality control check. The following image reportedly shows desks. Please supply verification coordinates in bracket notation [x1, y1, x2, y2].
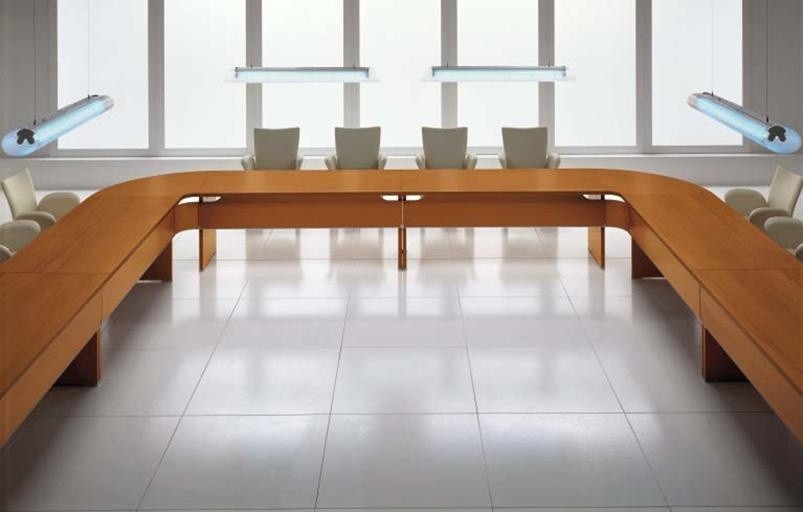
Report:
[0, 269, 105, 449]
[3, 199, 179, 383]
[630, 196, 803, 378]
[698, 266, 803, 447]
[83, 168, 705, 273]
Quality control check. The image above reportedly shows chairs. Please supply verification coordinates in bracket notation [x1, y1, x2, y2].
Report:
[1, 192, 40, 267]
[498, 127, 561, 169]
[4, 171, 79, 227]
[724, 165, 802, 259]
[415, 127, 478, 170]
[240, 127, 304, 171]
[325, 126, 387, 170]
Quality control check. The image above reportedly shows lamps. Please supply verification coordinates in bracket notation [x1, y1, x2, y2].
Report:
[1, 0, 114, 157]
[430, 65, 568, 83]
[234, 65, 371, 84]
[686, 0, 803, 154]
